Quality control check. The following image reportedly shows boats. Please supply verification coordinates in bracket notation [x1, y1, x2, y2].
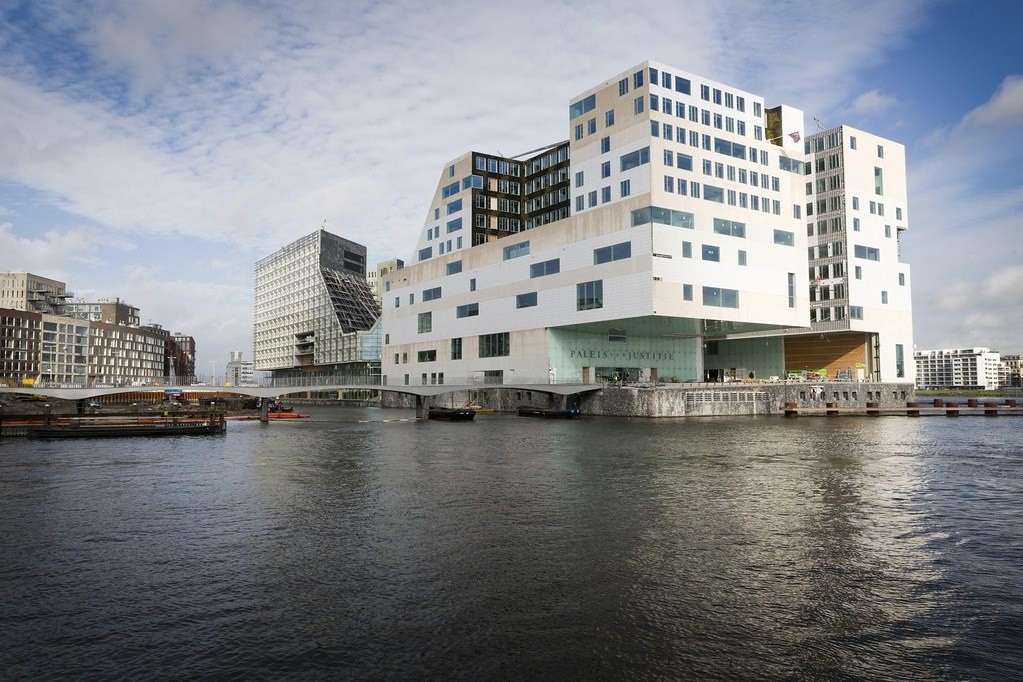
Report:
[428, 405, 476, 421]
[516, 405, 583, 420]
[27, 403, 228, 439]
